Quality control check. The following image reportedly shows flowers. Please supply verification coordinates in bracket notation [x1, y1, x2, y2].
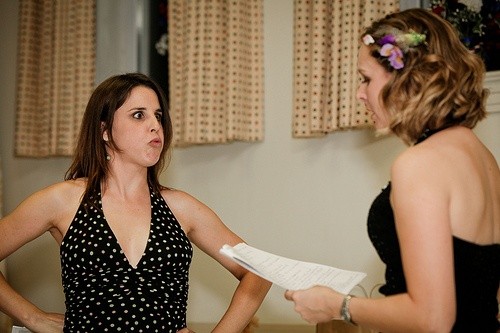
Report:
[376, 28, 426, 70]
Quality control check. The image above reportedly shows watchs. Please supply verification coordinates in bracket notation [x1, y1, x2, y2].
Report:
[340, 294, 358, 327]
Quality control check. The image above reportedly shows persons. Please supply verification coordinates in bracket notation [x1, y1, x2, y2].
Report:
[284, 8, 500, 333]
[0, 73, 273, 333]
